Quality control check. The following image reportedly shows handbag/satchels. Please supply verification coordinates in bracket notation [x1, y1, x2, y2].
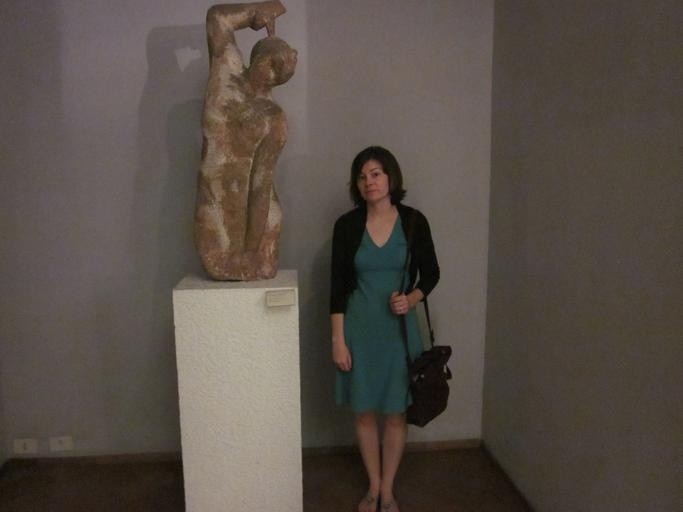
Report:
[406, 346, 451, 427]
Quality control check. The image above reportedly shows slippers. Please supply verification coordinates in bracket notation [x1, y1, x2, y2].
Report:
[380, 499, 399, 512]
[359, 495, 378, 512]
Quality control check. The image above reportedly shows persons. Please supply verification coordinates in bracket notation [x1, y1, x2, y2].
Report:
[195, 0, 300, 281]
[329, 141, 442, 511]
